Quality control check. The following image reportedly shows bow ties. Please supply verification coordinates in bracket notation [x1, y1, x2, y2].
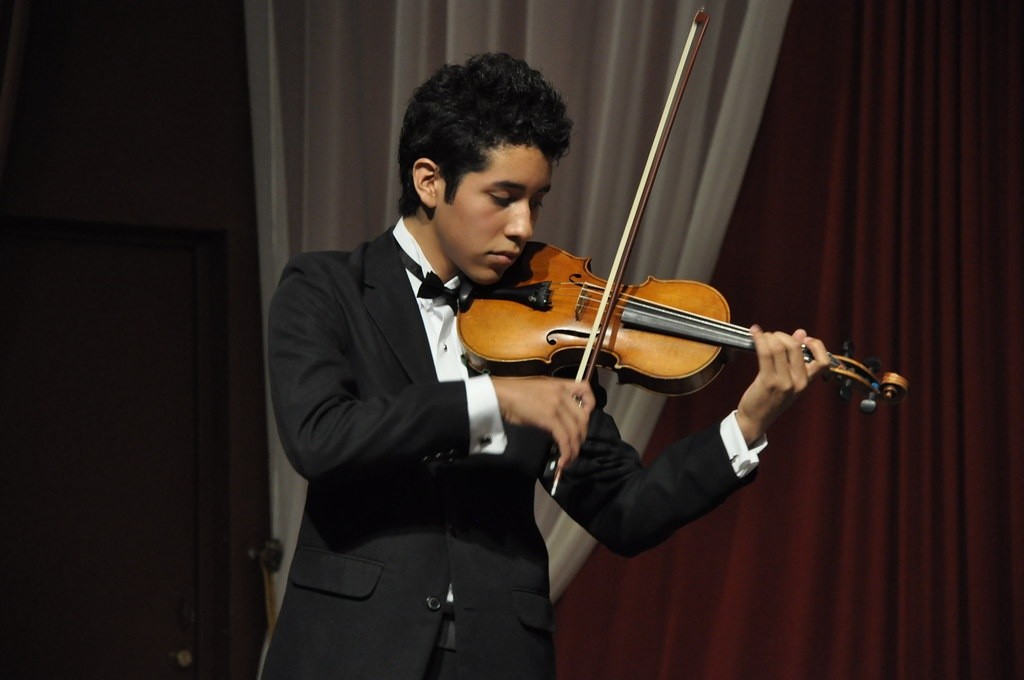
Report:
[392, 234, 461, 316]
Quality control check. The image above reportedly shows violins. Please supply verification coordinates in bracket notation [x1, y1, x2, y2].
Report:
[454, 237, 909, 415]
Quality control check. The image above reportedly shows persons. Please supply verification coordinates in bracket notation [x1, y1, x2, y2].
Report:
[256, 51, 832, 679]
